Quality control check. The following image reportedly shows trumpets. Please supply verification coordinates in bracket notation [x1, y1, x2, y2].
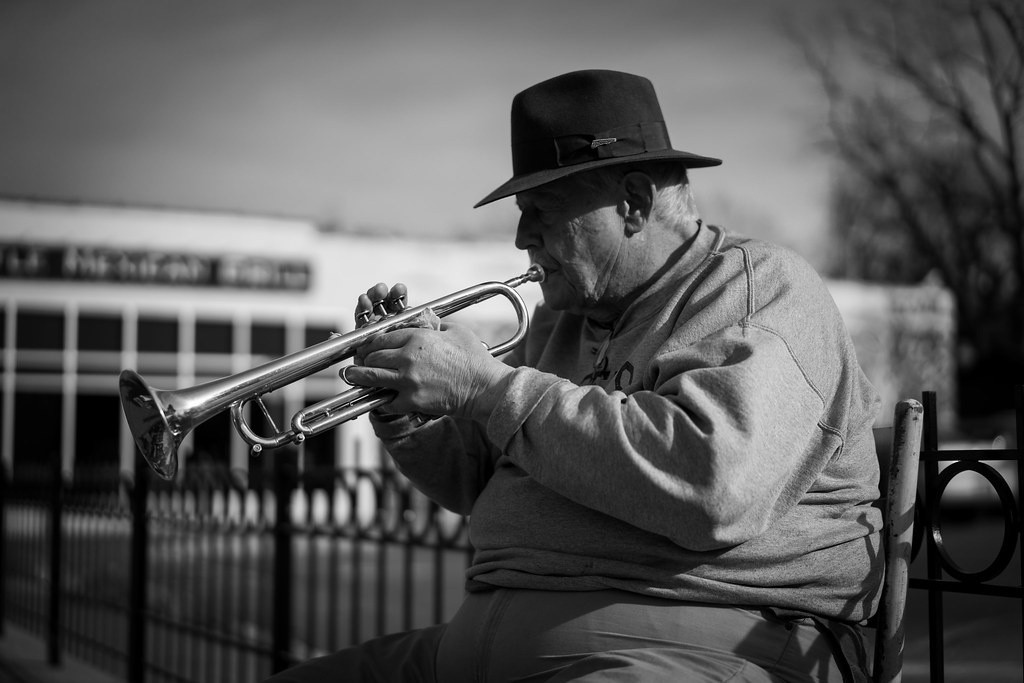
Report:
[116, 260, 547, 482]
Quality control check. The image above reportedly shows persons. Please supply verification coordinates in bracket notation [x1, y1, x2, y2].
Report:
[261, 67, 886, 683]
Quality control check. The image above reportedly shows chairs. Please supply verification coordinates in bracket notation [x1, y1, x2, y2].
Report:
[856, 399, 924, 683]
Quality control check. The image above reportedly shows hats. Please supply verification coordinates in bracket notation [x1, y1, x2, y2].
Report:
[471, 69, 722, 209]
